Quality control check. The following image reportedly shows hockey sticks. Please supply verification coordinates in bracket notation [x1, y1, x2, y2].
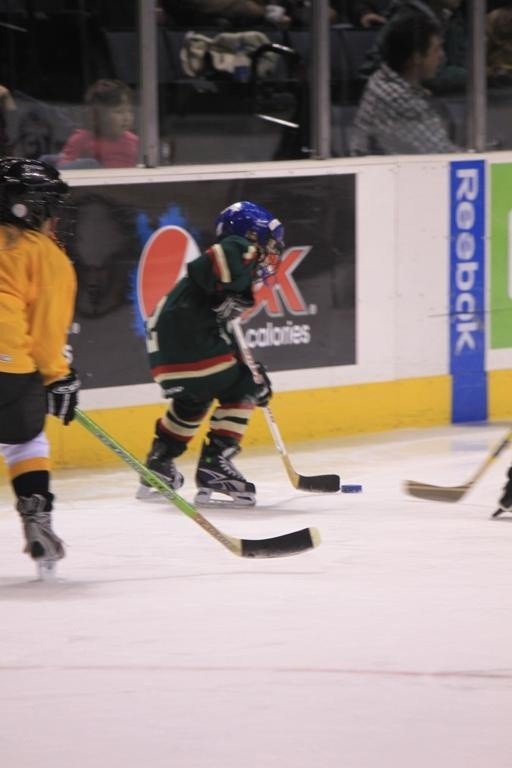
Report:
[231, 323, 341, 493]
[403, 431, 512, 504]
[70, 408, 322, 560]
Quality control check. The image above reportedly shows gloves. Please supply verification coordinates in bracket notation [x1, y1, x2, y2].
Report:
[249, 362, 271, 408]
[45, 368, 80, 426]
[216, 296, 244, 324]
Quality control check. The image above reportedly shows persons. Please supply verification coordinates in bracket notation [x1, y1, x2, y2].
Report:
[1, 1, 511, 166]
[138, 197, 286, 495]
[0, 157, 80, 563]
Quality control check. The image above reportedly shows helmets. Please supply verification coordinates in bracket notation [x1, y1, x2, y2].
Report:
[0, 157, 75, 245]
[215, 202, 285, 281]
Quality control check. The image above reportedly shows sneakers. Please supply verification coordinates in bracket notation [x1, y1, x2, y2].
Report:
[16, 494, 63, 561]
[195, 453, 257, 492]
[142, 454, 184, 488]
